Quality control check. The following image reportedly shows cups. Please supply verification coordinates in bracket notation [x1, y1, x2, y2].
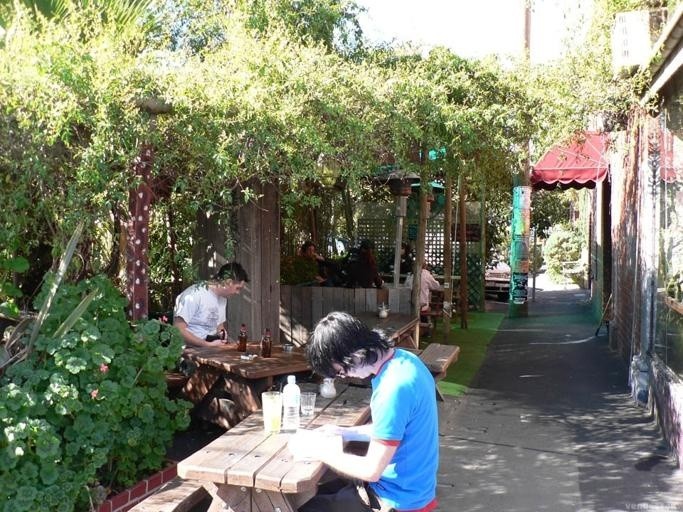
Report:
[283, 343, 293, 353]
[300, 392, 316, 415]
[260, 390, 284, 435]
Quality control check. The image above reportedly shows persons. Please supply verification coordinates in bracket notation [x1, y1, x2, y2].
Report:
[402, 258, 441, 339]
[296, 310, 438, 511]
[172, 261, 258, 435]
[285, 237, 415, 290]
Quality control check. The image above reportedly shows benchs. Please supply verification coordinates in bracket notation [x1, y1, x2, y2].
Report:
[378, 273, 462, 337]
[485, 272, 511, 294]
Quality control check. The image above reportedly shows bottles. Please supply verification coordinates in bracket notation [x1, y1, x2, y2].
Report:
[261, 327, 272, 357]
[237, 322, 248, 352]
[281, 374, 301, 432]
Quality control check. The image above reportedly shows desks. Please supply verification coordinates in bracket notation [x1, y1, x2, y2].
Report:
[119, 309, 461, 512]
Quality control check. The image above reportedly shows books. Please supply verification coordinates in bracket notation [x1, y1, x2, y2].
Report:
[286, 427, 343, 464]
